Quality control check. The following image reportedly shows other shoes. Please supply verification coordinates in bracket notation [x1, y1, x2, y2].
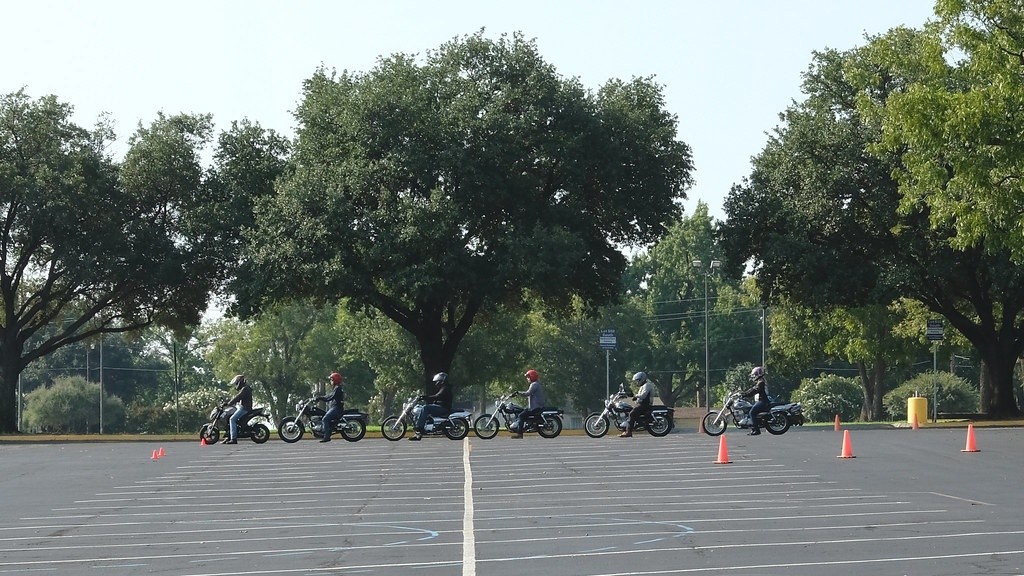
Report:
[406, 434, 421, 441]
[747, 429, 755, 436]
[219, 437, 230, 444]
[752, 430, 761, 435]
[226, 439, 237, 444]
[511, 433, 523, 439]
[617, 429, 632, 437]
[319, 436, 332, 443]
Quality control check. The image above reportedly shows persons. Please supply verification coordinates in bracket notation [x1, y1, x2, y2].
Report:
[220, 375, 252, 444]
[511, 370, 545, 438]
[738, 367, 769, 435]
[313, 373, 344, 442]
[617, 372, 654, 437]
[409, 372, 452, 440]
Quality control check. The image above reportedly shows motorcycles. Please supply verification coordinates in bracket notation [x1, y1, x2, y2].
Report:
[702, 385, 791, 437]
[380, 390, 473, 442]
[583, 382, 676, 439]
[198, 396, 271, 446]
[766, 393, 805, 427]
[473, 384, 564, 440]
[278, 390, 370, 444]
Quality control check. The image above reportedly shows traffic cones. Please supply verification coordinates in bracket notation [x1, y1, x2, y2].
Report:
[200, 438, 208, 446]
[960, 424, 981, 452]
[150, 449, 160, 460]
[910, 413, 921, 430]
[713, 435, 733, 465]
[833, 414, 842, 432]
[697, 415, 705, 434]
[836, 429, 858, 459]
[157, 447, 166, 456]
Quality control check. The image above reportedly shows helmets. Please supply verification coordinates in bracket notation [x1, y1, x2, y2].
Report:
[751, 366, 765, 382]
[525, 370, 539, 382]
[230, 375, 246, 390]
[632, 372, 647, 387]
[329, 373, 342, 385]
[432, 372, 448, 387]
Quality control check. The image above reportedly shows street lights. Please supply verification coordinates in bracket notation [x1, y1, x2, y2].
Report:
[85, 343, 95, 384]
[690, 260, 721, 430]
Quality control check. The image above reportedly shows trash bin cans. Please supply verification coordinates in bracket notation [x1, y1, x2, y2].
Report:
[907, 397, 927, 423]
